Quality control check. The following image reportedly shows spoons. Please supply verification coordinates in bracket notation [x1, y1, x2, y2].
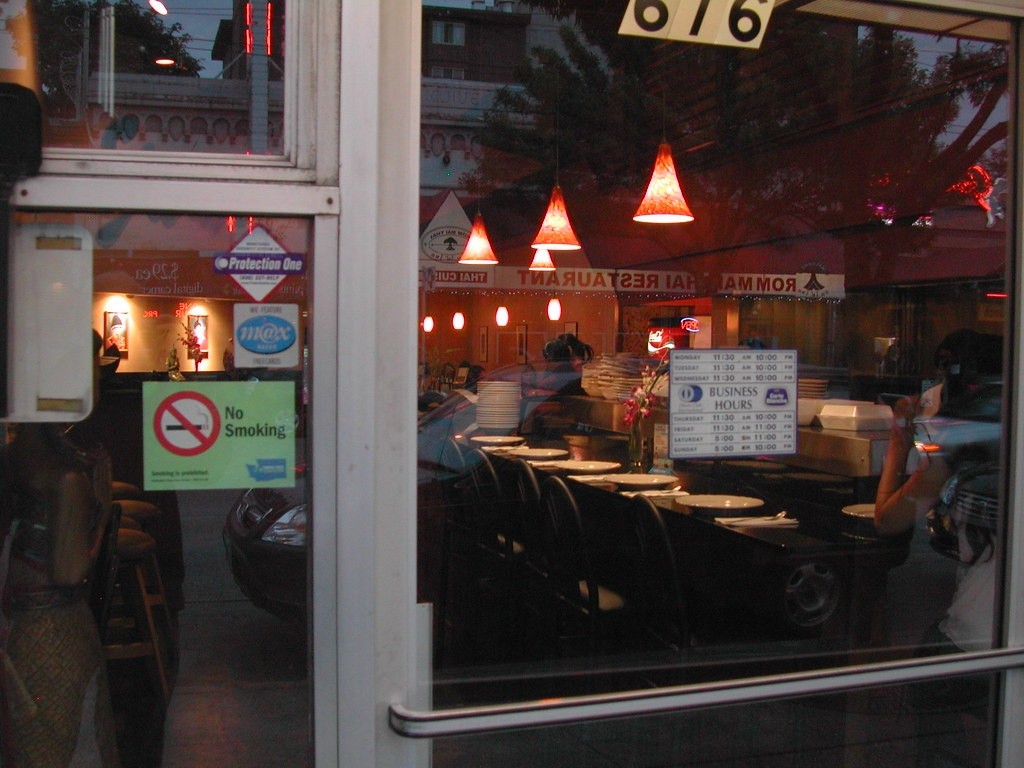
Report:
[724, 511, 786, 523]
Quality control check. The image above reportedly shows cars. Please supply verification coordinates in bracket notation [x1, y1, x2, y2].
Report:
[221, 322, 1006, 637]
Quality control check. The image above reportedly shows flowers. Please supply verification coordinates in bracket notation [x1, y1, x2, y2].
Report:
[177, 322, 204, 377]
[623, 337, 676, 428]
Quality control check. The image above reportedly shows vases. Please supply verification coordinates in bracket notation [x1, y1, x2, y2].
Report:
[628, 419, 643, 466]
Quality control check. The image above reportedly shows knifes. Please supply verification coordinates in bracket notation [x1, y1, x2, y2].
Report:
[726, 523, 799, 527]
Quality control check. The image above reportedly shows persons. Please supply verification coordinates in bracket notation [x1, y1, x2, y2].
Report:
[557, 333, 594, 372]
[524, 341, 584, 397]
[874, 329, 1002, 768]
[465, 365, 486, 396]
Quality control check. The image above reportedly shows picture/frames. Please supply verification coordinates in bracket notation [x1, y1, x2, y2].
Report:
[480, 326, 488, 362]
[564, 322, 578, 337]
[516, 323, 527, 363]
[187, 315, 208, 353]
[104, 311, 129, 353]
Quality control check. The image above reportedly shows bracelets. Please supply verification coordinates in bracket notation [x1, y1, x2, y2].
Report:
[533, 389, 537, 396]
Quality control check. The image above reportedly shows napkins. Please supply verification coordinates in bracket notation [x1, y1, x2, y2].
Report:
[528, 459, 571, 469]
[482, 446, 528, 453]
[621, 490, 689, 498]
[715, 516, 800, 528]
[567, 474, 617, 484]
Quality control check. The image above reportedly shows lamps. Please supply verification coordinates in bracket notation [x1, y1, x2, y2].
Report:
[424, 49, 694, 332]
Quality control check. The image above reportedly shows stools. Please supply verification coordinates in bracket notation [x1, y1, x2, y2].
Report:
[94, 482, 175, 702]
[433, 438, 803, 768]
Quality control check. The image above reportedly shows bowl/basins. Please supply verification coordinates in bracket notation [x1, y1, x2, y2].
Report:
[798, 399, 816, 426]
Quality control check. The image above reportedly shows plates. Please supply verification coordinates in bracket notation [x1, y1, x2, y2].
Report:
[676, 495, 764, 512]
[476, 381, 521, 436]
[603, 474, 679, 488]
[842, 504, 876, 518]
[470, 437, 524, 445]
[553, 460, 621, 473]
[509, 449, 568, 460]
[581, 353, 643, 399]
[798, 379, 830, 401]
